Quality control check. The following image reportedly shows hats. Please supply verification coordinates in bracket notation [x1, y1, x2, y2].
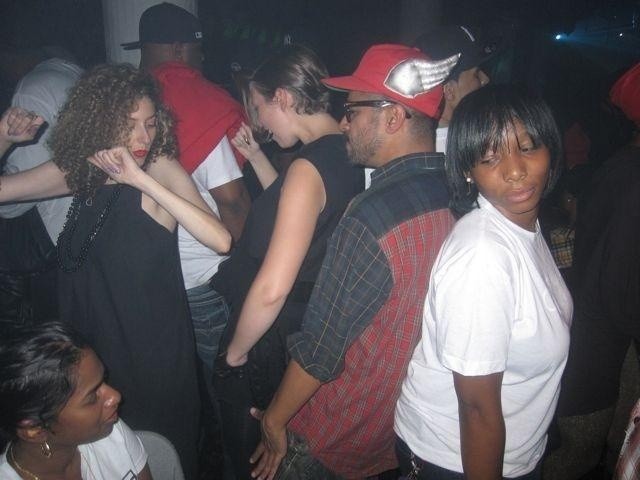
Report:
[411, 24, 497, 83]
[120, 2, 205, 47]
[320, 42, 462, 120]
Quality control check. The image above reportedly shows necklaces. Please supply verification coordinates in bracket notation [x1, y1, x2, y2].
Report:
[56, 162, 146, 273]
[11, 444, 40, 477]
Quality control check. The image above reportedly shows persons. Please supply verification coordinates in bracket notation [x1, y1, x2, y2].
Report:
[2, 2, 639, 478]
